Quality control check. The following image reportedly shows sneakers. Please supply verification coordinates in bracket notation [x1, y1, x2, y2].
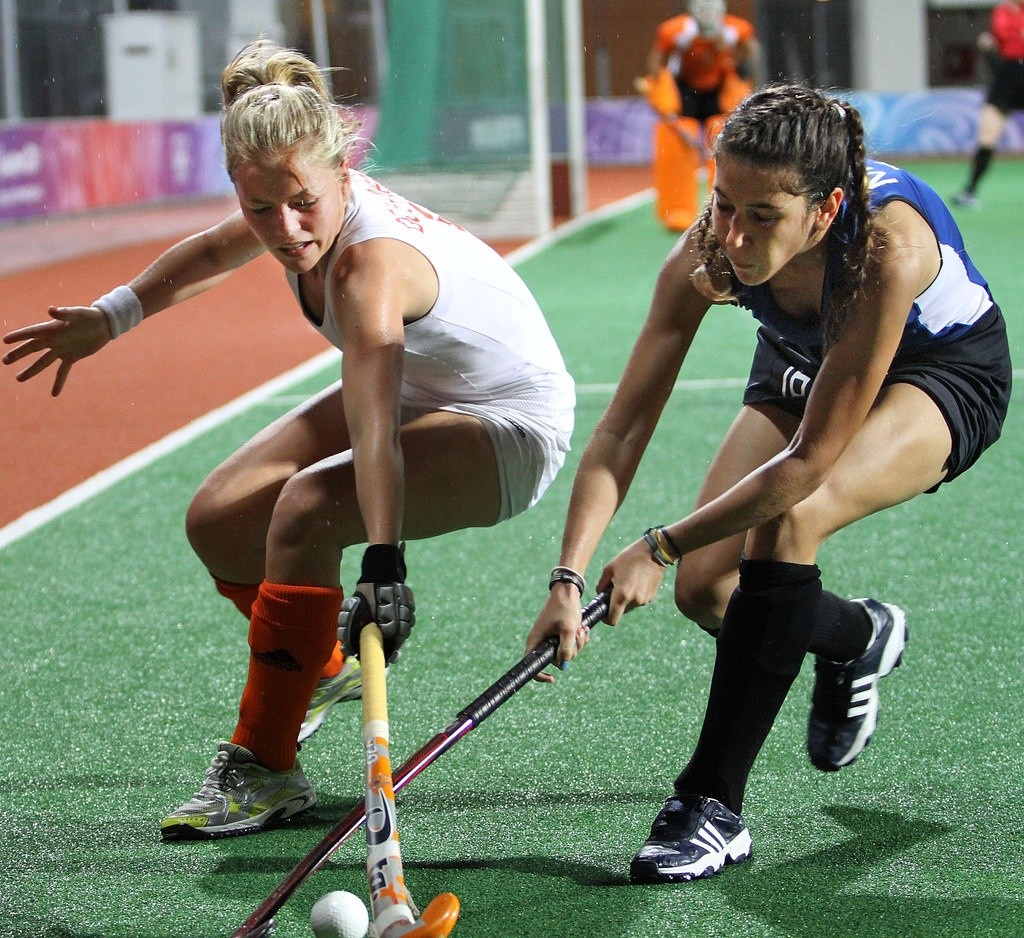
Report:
[160, 741, 318, 839]
[631, 794, 754, 884]
[807, 600, 910, 773]
[295, 644, 390, 746]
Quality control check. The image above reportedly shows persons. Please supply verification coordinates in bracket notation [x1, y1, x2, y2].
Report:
[523, 83, 1014, 885]
[646, 0, 765, 233]
[950, 0, 1024, 208]
[2, 32, 574, 838]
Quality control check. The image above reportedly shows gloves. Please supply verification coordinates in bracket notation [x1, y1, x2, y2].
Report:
[337, 539, 416, 670]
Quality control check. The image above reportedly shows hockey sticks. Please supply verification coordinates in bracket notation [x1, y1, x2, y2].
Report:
[221, 585, 613, 938]
[355, 625, 461, 937]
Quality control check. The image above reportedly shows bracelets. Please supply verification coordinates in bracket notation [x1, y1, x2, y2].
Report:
[549, 566, 586, 598]
[644, 525, 682, 568]
[91, 285, 144, 340]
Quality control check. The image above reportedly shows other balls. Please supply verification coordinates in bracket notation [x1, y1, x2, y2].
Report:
[310, 889, 370, 938]
